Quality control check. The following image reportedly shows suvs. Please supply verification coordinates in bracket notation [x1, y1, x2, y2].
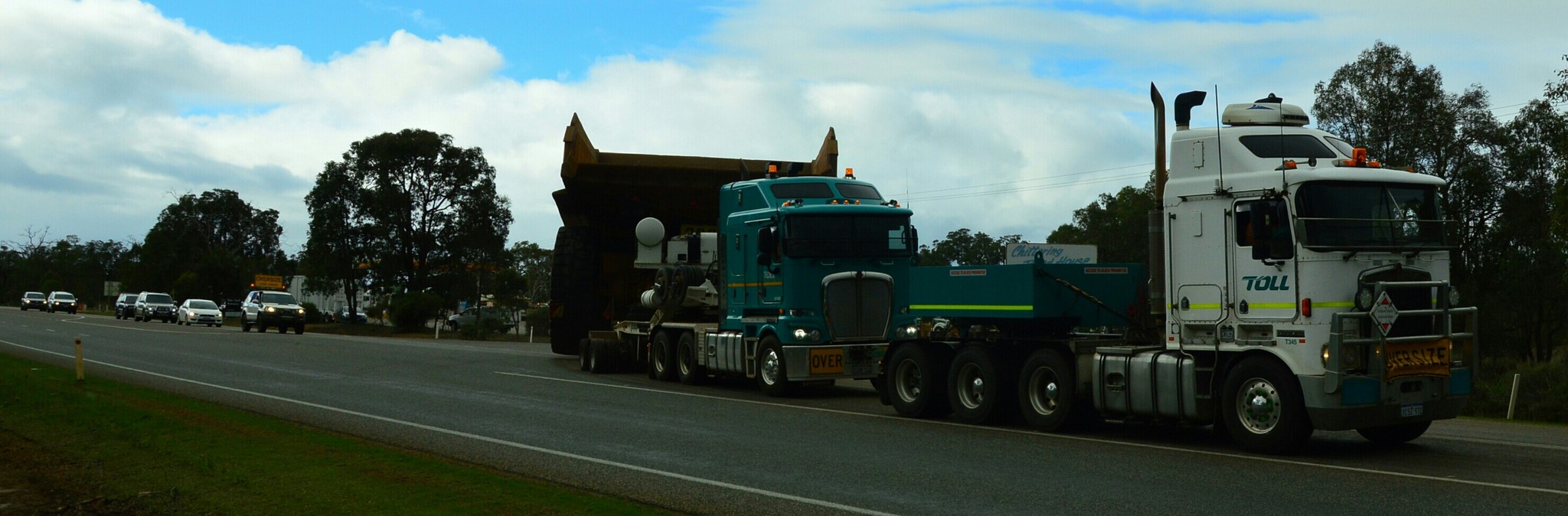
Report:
[114, 293, 140, 321]
[47, 291, 78, 314]
[240, 285, 306, 334]
[134, 291, 180, 323]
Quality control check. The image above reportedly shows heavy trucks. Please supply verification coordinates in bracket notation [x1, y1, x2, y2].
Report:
[572, 164, 918, 398]
[881, 82, 1480, 455]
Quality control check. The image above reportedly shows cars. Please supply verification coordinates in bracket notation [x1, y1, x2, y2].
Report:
[333, 306, 368, 322]
[178, 298, 224, 327]
[21, 292, 48, 311]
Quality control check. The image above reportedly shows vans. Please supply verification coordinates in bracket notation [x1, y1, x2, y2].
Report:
[444, 306, 517, 334]
